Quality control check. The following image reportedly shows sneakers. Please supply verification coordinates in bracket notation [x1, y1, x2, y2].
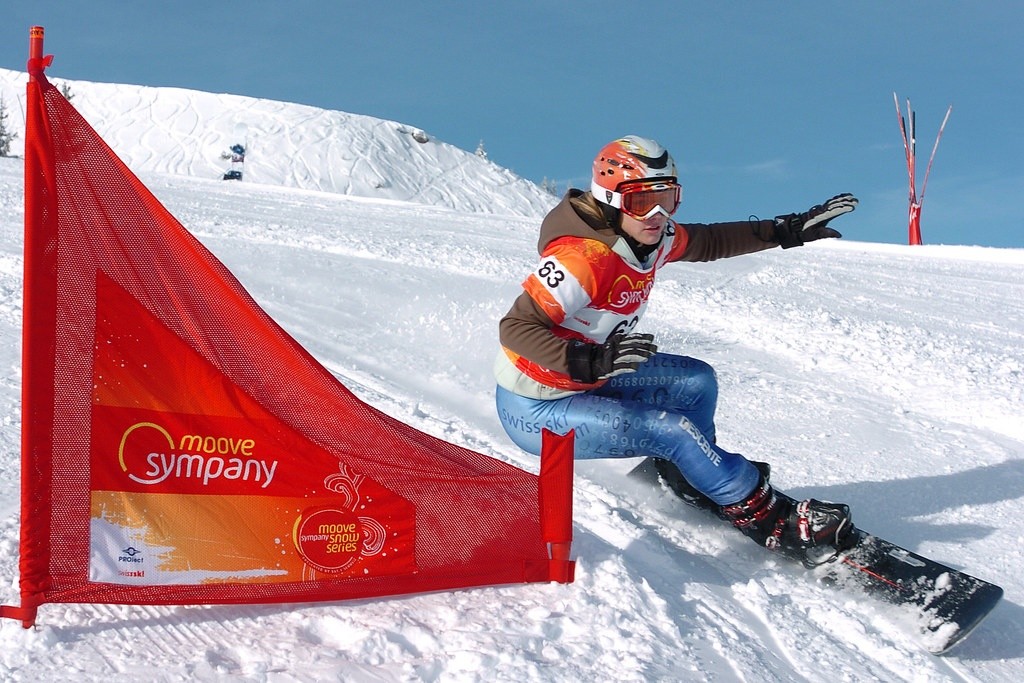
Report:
[717, 471, 852, 551]
[651, 454, 771, 512]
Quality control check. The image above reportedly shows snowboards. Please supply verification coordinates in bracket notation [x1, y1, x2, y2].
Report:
[620, 438, 1005, 657]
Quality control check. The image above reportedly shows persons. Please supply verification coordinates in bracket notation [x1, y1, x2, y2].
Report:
[495, 134, 860, 570]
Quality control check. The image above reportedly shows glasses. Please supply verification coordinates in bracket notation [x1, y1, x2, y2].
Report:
[620, 184, 683, 221]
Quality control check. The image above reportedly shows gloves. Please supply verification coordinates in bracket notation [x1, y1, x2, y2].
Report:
[565, 333, 657, 384]
[775, 192, 859, 249]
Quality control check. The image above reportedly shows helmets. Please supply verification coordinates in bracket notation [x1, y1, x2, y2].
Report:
[592, 134, 678, 192]
